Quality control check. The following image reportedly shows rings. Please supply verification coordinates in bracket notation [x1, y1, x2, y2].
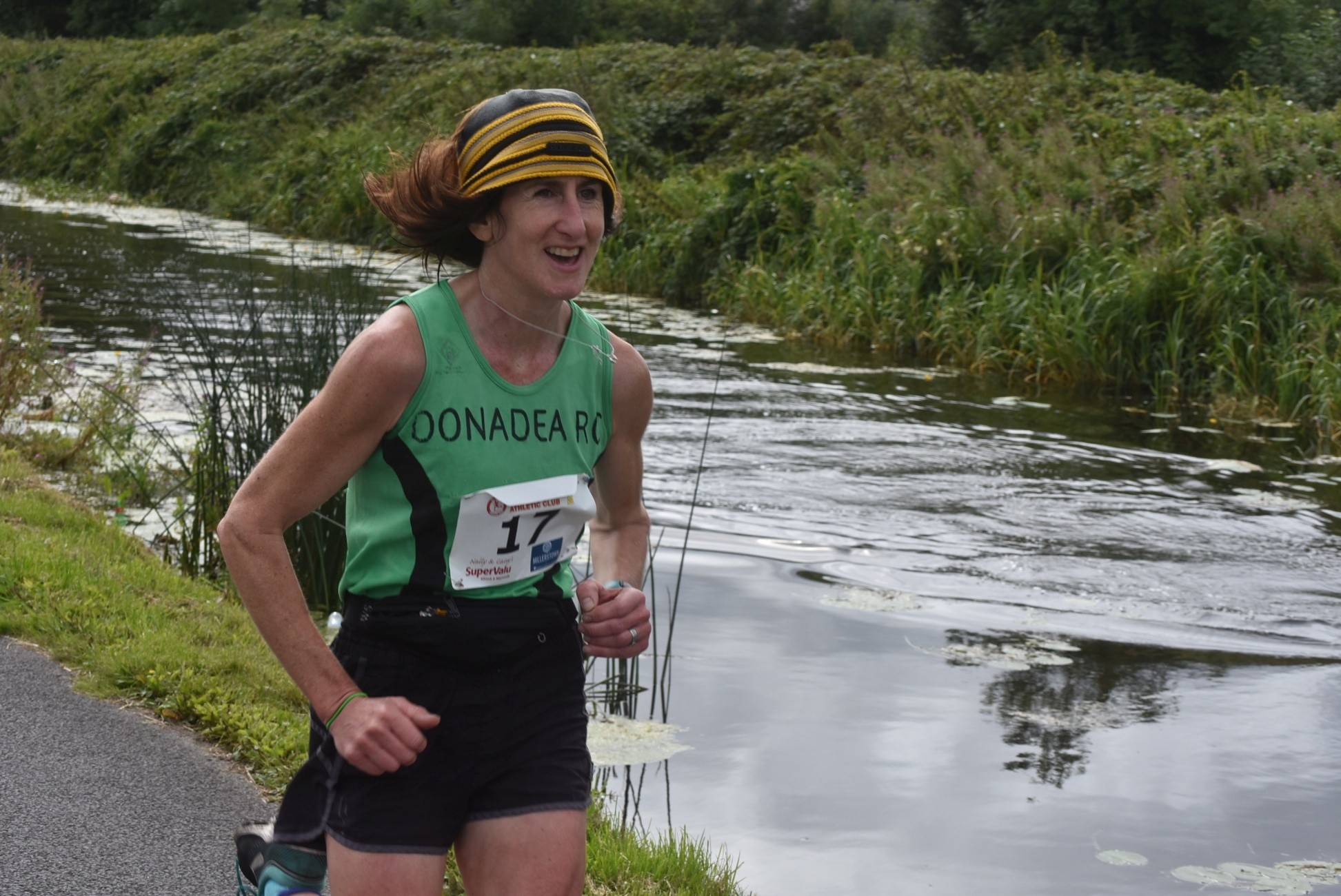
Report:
[630, 628, 638, 647]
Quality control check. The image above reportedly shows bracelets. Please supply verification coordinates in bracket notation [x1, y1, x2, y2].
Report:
[325, 692, 368, 731]
[603, 580, 632, 591]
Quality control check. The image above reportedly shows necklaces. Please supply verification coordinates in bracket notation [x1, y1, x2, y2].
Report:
[480, 284, 618, 363]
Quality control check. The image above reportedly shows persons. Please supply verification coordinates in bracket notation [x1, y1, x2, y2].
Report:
[214, 87, 654, 896]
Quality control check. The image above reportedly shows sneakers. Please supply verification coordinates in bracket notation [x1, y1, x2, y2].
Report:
[233, 819, 327, 896]
